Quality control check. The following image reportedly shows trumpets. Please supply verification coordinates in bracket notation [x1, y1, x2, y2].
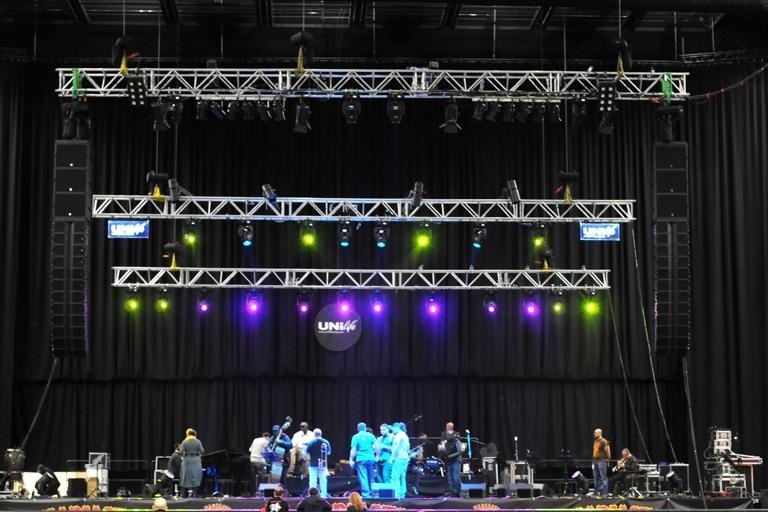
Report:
[611, 459, 628, 472]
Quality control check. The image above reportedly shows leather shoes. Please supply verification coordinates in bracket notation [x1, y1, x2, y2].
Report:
[411, 487, 419, 495]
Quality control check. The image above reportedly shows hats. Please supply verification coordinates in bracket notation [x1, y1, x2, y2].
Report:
[272, 425, 280, 431]
[37, 465, 44, 469]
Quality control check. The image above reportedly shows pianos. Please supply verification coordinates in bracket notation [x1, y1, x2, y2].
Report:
[201, 447, 260, 497]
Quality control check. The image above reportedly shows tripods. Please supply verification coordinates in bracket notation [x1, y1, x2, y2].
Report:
[86, 462, 109, 499]
[623, 478, 643, 498]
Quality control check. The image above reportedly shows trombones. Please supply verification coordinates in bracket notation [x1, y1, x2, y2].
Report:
[318, 443, 327, 498]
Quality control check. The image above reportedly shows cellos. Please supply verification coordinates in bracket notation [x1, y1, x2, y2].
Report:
[264, 416, 292, 483]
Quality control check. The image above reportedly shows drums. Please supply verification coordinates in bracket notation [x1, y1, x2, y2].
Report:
[422, 456, 447, 479]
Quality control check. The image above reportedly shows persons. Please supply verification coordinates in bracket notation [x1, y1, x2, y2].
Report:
[263, 487, 289, 511]
[592, 428, 639, 499]
[152, 498, 168, 511]
[346, 492, 368, 511]
[297, 488, 332, 511]
[168, 428, 203, 499]
[248, 422, 332, 497]
[340, 422, 463, 501]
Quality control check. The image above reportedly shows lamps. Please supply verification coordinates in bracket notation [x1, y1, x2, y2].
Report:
[238, 220, 254, 247]
[416, 222, 433, 247]
[578, 289, 599, 315]
[154, 287, 171, 314]
[530, 222, 549, 247]
[550, 289, 567, 316]
[373, 222, 390, 248]
[196, 288, 215, 313]
[335, 221, 353, 248]
[411, 181, 424, 208]
[471, 223, 488, 249]
[168, 177, 181, 205]
[369, 290, 387, 315]
[182, 218, 201, 247]
[336, 290, 354, 315]
[424, 290, 442, 316]
[296, 289, 313, 315]
[123, 75, 619, 132]
[482, 291, 498, 314]
[245, 288, 264, 314]
[506, 179, 522, 205]
[261, 183, 278, 204]
[123, 288, 142, 314]
[521, 290, 541, 316]
[298, 220, 317, 247]
